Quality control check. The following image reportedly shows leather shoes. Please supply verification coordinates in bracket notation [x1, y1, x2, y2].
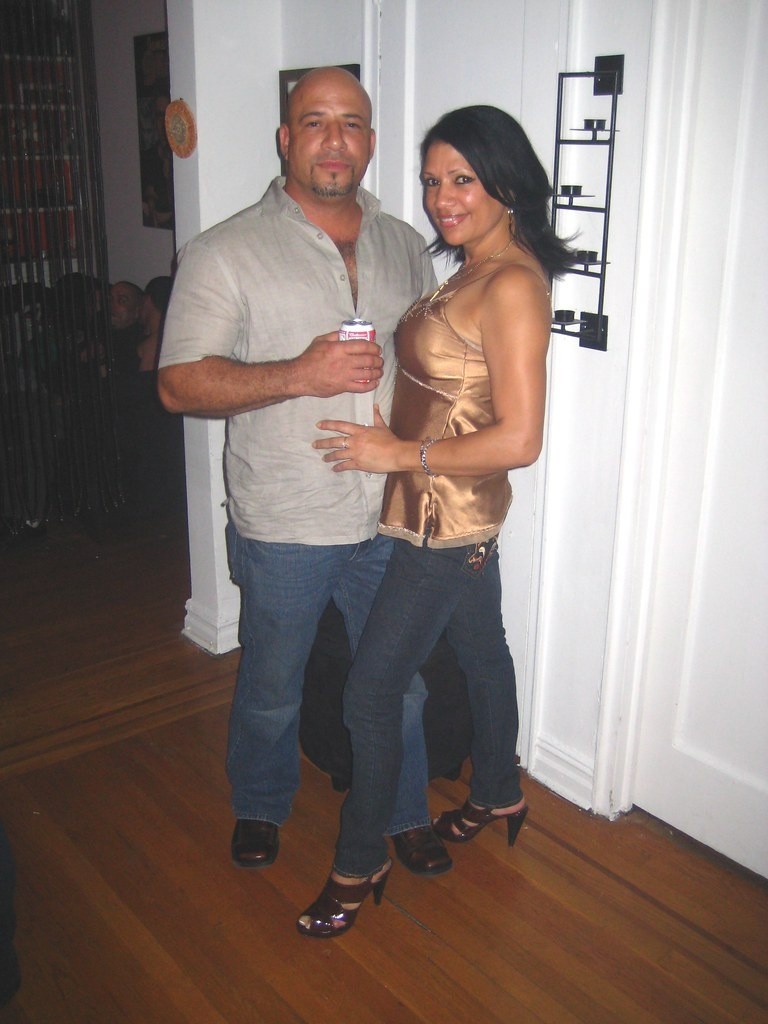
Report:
[230, 819, 279, 868]
[392, 826, 453, 876]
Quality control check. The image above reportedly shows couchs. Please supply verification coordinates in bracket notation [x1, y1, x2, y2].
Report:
[0, 271, 187, 545]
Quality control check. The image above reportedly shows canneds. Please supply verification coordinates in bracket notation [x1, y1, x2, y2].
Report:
[338, 318, 376, 384]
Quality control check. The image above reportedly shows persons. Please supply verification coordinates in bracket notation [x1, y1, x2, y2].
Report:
[56, 276, 177, 451]
[298, 106, 576, 938]
[159, 65, 455, 875]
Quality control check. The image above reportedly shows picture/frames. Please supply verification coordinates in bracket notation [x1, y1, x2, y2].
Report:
[278, 63, 360, 178]
[133, 31, 174, 230]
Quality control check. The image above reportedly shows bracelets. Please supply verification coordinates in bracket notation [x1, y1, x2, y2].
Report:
[421, 438, 434, 475]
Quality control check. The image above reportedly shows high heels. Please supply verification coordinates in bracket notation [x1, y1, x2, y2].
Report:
[297, 858, 391, 939]
[432, 796, 529, 848]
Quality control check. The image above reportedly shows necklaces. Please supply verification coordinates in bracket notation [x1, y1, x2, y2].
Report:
[429, 240, 513, 302]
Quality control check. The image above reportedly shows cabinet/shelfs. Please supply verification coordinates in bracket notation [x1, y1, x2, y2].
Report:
[0, 51, 86, 264]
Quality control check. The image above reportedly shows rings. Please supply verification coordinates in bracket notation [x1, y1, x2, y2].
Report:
[344, 437, 347, 448]
